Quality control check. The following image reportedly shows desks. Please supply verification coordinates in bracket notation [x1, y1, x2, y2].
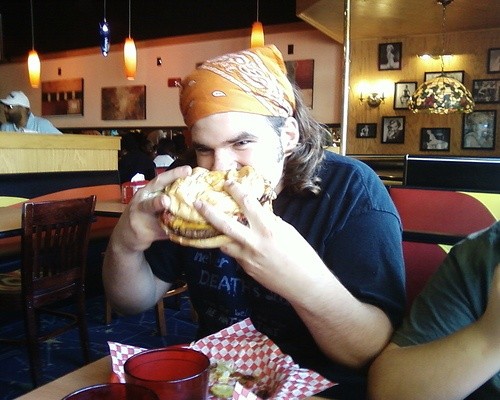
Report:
[10, 355, 332, 400]
[94, 199, 128, 218]
[0, 195, 98, 239]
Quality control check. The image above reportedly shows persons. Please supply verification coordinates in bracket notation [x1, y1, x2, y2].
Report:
[361, 125, 369, 136]
[386, 44, 395, 67]
[102, 45, 406, 400]
[427, 130, 448, 149]
[464, 112, 492, 149]
[368, 222, 500, 400]
[385, 119, 403, 143]
[479, 80, 497, 102]
[400, 84, 410, 104]
[0, 91, 62, 135]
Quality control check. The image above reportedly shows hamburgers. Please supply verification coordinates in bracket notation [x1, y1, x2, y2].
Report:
[160, 165, 277, 249]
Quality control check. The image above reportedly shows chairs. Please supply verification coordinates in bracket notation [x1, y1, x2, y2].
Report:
[0, 194, 200, 390]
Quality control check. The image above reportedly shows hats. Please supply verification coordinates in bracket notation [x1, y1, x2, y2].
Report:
[0, 91, 31, 109]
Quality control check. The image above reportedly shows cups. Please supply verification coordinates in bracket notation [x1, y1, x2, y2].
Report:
[60, 382, 161, 400]
[123, 346, 210, 400]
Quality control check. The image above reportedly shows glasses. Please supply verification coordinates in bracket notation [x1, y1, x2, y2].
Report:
[6, 105, 14, 110]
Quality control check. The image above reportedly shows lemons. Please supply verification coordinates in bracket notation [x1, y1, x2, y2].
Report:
[210, 384, 234, 398]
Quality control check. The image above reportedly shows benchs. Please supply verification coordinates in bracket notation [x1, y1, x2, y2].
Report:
[0, 169, 122, 277]
[385, 154, 500, 318]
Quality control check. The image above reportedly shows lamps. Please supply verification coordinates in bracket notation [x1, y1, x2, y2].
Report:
[28, 0, 41, 88]
[406, 0, 475, 114]
[353, 78, 395, 108]
[124, 0, 137, 81]
[417, 46, 453, 62]
[99, 0, 110, 57]
[250, 0, 264, 48]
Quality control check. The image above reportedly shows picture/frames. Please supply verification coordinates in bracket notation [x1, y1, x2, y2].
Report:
[357, 42, 500, 152]
